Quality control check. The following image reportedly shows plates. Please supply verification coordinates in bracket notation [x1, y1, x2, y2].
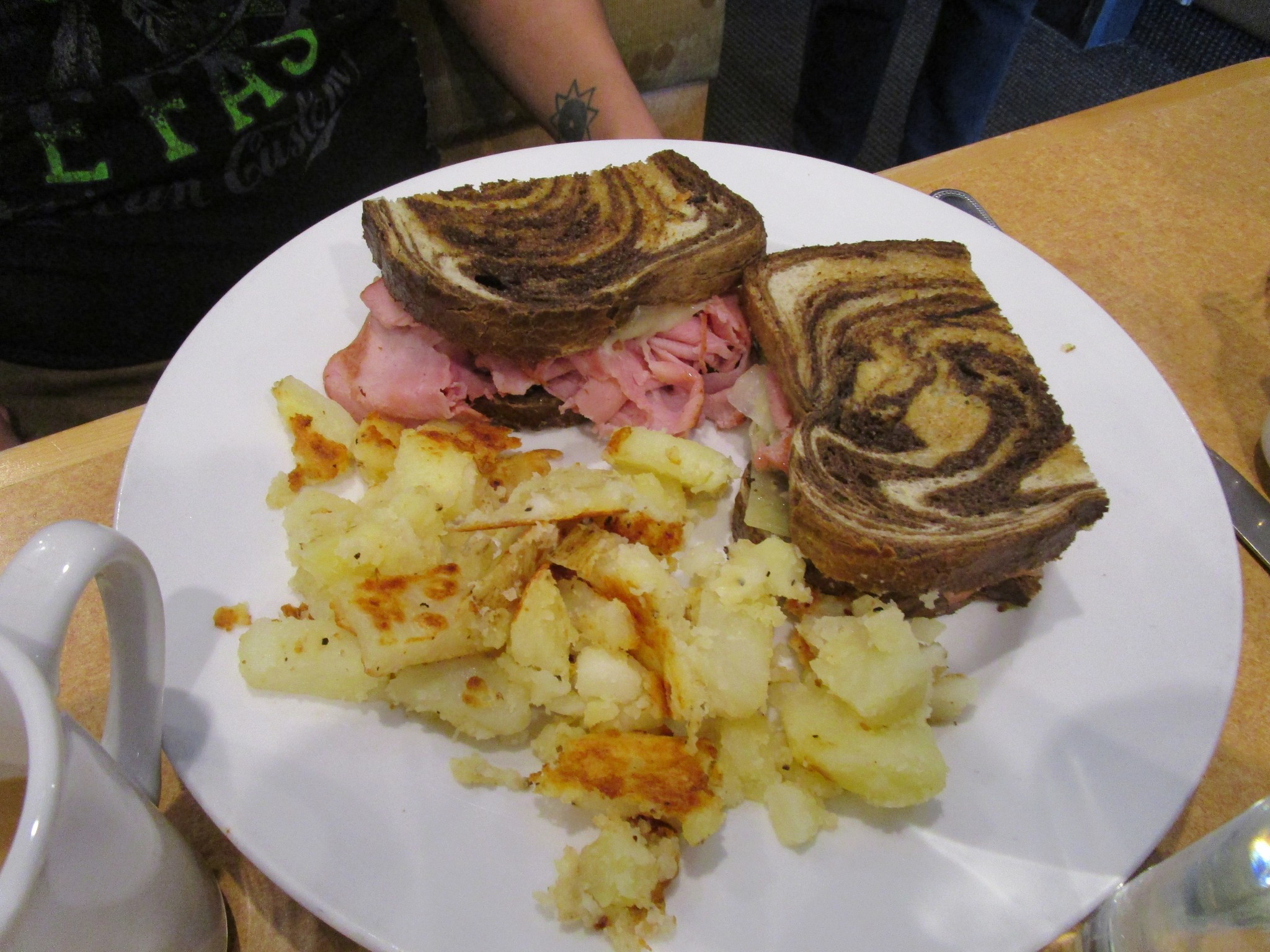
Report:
[114, 139, 1243, 951]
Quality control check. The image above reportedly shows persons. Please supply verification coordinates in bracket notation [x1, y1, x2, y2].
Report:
[0, 0, 664, 446]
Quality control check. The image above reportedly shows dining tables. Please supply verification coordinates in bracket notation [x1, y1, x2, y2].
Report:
[0, 52, 1270, 952]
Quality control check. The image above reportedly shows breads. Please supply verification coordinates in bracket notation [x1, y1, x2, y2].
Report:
[459, 386, 590, 430]
[730, 461, 1042, 619]
[741, 242, 1108, 604]
[360, 149, 766, 358]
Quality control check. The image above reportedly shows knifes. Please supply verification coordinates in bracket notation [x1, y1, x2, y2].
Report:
[931, 189, 1270, 569]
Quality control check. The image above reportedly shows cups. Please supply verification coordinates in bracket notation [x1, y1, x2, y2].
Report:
[1079, 791, 1270, 952]
[0, 519, 228, 952]
